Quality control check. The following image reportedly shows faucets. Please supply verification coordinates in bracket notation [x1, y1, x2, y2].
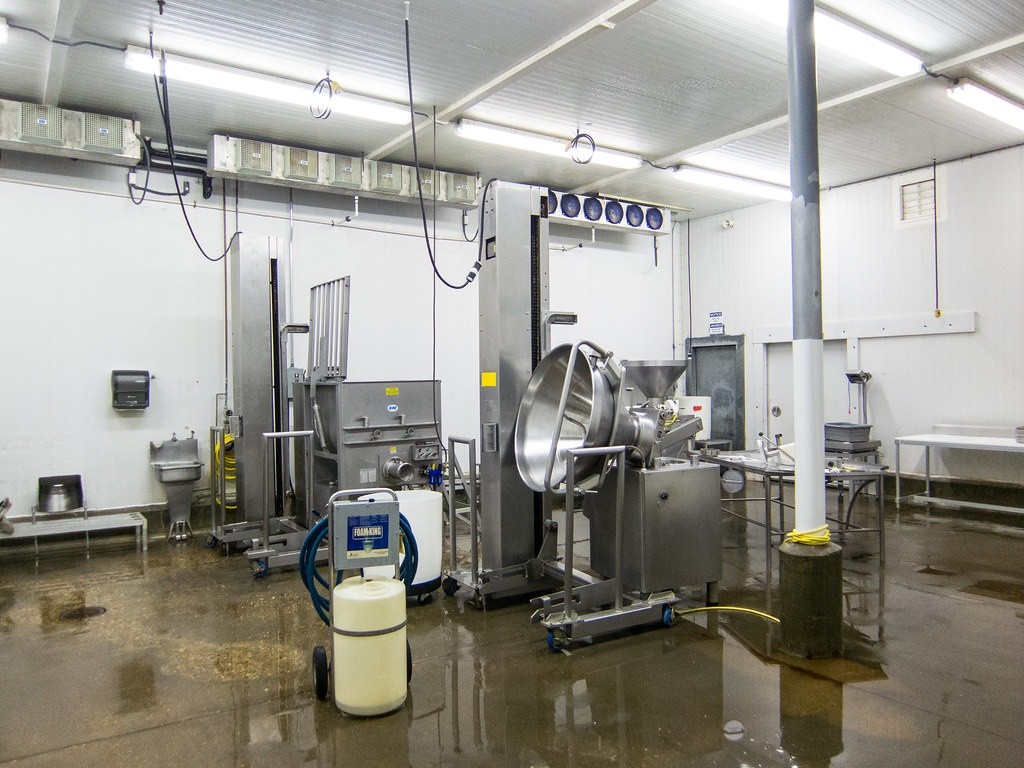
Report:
[171, 432, 178, 442]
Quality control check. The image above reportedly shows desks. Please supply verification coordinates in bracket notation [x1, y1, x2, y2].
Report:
[893, 433, 1024, 539]
[700, 446, 892, 615]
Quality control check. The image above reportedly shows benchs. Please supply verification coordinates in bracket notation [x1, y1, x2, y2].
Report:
[0, 511, 147, 552]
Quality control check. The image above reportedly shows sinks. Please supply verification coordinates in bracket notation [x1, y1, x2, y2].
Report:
[151, 438, 205, 483]
